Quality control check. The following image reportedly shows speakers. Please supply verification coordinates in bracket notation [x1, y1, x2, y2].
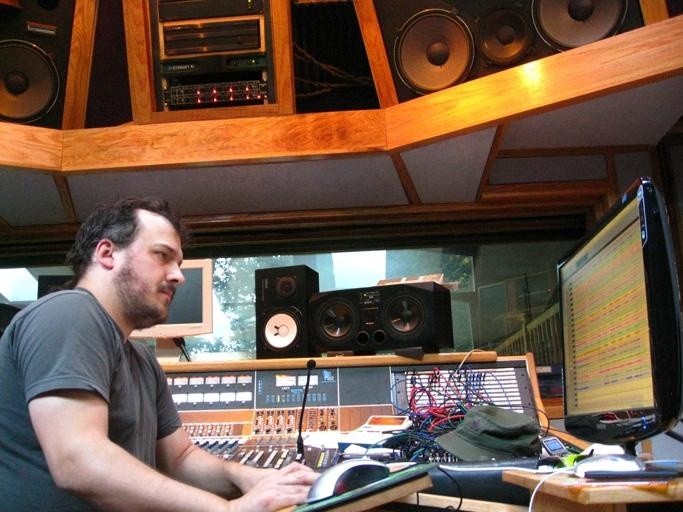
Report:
[38, 274, 77, 301]
[255, 265, 322, 359]
[308, 282, 454, 355]
[372, 0, 645, 104]
[0, 0, 76, 130]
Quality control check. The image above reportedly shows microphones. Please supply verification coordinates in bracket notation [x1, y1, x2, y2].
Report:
[297, 360, 316, 457]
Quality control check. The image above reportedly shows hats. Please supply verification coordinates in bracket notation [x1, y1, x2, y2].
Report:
[434, 405, 541, 462]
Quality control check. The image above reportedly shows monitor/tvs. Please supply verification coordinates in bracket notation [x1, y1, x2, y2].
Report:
[128, 258, 213, 363]
[556, 176, 683, 457]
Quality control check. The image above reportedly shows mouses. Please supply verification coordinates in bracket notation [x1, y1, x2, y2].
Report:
[306, 458, 390, 504]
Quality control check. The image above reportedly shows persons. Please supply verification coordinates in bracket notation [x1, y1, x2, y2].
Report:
[0, 198, 318, 512]
